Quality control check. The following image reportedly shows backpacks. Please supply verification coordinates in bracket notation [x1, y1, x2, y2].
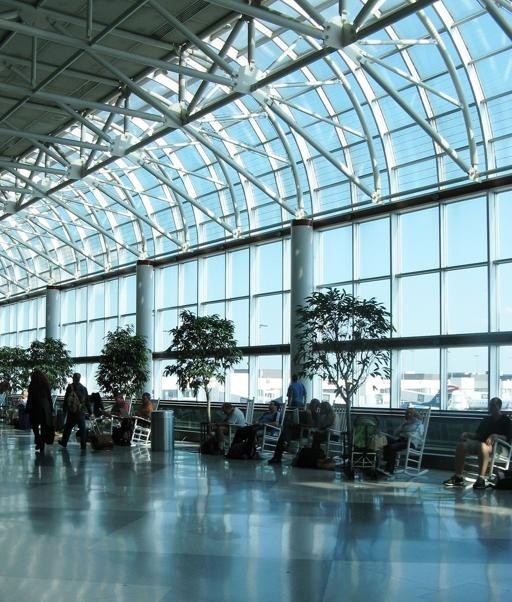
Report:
[239, 430, 257, 459]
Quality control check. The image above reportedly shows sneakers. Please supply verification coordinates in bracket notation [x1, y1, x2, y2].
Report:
[36, 444, 44, 451]
[473, 480, 485, 489]
[58, 440, 66, 447]
[377, 468, 392, 476]
[443, 476, 464, 486]
[81, 441, 86, 449]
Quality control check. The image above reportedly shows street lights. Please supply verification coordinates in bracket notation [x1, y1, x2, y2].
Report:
[258, 324, 269, 379]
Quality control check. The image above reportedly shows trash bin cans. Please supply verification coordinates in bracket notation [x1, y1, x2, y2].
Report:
[151, 410, 175, 452]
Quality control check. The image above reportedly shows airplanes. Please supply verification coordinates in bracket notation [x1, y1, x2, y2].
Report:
[401, 382, 459, 411]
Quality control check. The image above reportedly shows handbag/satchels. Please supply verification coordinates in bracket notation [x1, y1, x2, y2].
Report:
[292, 447, 336, 469]
[200, 438, 225, 455]
[112, 426, 131, 446]
[492, 471, 511, 489]
[368, 436, 388, 449]
[65, 384, 80, 413]
[42, 423, 55, 444]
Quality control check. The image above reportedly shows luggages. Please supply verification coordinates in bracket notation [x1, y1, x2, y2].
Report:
[87, 413, 113, 450]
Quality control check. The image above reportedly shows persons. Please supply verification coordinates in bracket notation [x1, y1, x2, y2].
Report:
[6, 397, 26, 425]
[26, 367, 55, 451]
[381, 408, 424, 480]
[111, 393, 129, 416]
[442, 397, 511, 489]
[214, 374, 340, 464]
[129, 392, 155, 441]
[58, 372, 90, 450]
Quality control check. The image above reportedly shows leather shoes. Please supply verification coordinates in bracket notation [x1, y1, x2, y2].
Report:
[268, 457, 282, 463]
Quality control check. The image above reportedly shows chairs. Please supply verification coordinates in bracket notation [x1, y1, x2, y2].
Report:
[219, 397, 433, 477]
[1, 385, 161, 446]
[449, 412, 511, 491]
